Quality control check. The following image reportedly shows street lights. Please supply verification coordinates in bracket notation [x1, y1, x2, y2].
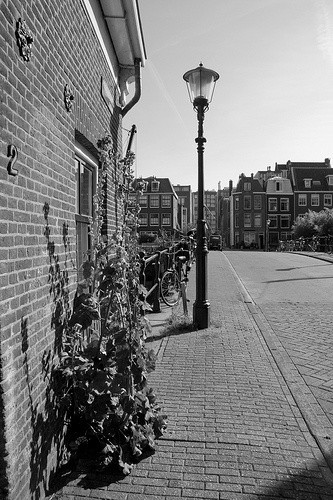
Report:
[183, 62, 221, 327]
[265, 220, 271, 252]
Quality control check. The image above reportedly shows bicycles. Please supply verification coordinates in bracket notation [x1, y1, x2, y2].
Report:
[157, 248, 188, 307]
[277, 234, 333, 254]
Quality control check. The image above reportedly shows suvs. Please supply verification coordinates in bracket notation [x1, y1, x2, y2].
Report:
[208, 235, 222, 251]
